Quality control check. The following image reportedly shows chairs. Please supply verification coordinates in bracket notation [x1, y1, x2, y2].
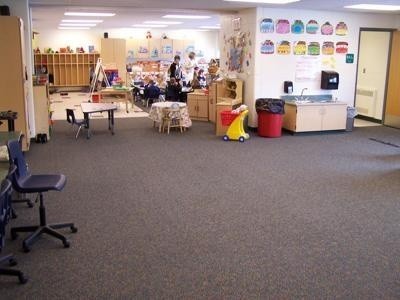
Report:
[162, 102, 182, 135]
[0, 139, 77, 285]
[66, 109, 90, 138]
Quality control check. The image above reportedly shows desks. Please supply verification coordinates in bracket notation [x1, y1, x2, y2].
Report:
[0, 132, 22, 184]
[99, 87, 135, 113]
[0, 111, 18, 131]
[149, 102, 192, 133]
[80, 103, 117, 139]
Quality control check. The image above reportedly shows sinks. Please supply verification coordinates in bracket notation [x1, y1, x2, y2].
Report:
[295, 101, 313, 104]
[326, 101, 341, 104]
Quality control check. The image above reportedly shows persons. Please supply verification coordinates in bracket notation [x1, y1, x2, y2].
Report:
[143, 52, 204, 102]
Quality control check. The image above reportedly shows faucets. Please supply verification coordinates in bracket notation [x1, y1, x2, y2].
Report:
[300, 88, 308, 99]
[335, 97, 338, 101]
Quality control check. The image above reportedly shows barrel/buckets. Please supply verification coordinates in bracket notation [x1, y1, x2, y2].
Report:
[92, 94, 100, 103]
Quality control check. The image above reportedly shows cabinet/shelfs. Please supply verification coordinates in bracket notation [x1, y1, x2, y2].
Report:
[187, 79, 243, 136]
[34, 53, 101, 88]
[282, 104, 347, 133]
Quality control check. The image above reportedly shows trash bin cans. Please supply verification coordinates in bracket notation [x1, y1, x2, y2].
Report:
[342, 106, 356, 132]
[256, 98, 285, 138]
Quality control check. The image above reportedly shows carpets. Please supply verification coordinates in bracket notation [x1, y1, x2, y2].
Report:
[50, 92, 149, 120]
